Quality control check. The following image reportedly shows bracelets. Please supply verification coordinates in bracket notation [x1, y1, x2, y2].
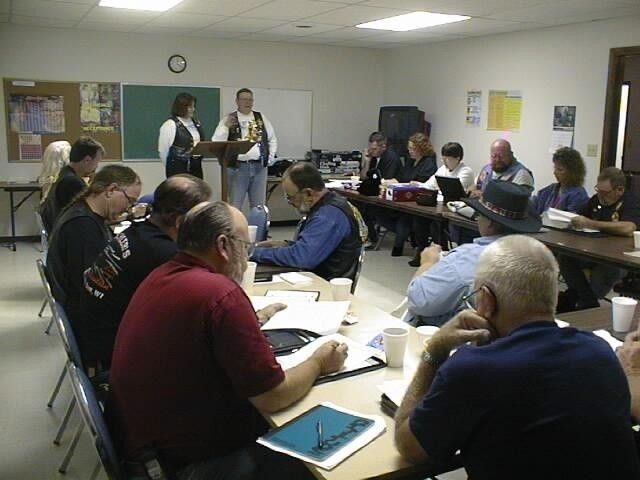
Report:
[420, 351, 442, 369]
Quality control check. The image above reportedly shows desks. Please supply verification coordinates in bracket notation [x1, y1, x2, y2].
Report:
[0, 180, 42, 251]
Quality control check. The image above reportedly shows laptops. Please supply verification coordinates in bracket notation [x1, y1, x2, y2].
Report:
[435, 176, 471, 201]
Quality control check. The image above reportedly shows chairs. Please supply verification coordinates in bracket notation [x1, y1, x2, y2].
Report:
[35, 208, 130, 480]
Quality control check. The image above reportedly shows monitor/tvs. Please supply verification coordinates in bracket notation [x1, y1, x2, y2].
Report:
[378, 106, 431, 153]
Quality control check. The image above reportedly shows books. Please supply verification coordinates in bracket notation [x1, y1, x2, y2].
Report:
[256, 402, 387, 471]
[380, 379, 410, 419]
[540, 207, 600, 233]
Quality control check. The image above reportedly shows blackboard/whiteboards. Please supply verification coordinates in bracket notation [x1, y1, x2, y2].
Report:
[120, 82, 222, 162]
[222, 86, 313, 161]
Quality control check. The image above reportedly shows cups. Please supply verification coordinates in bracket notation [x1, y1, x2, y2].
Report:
[611, 296, 638, 332]
[382, 327, 406, 368]
[633, 231, 640, 248]
[351, 176, 360, 186]
[242, 262, 257, 292]
[330, 278, 353, 302]
[417, 325, 441, 356]
[248, 225, 258, 243]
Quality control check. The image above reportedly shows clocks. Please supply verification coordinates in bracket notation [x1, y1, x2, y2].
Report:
[168, 54, 187, 73]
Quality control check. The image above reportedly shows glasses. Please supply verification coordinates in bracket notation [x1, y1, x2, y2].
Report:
[235, 97, 253, 102]
[407, 146, 418, 151]
[122, 189, 139, 206]
[227, 235, 258, 257]
[285, 189, 301, 200]
[440, 156, 457, 163]
[463, 285, 493, 312]
[594, 185, 621, 197]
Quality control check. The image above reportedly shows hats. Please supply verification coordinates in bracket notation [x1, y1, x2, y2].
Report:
[461, 179, 542, 233]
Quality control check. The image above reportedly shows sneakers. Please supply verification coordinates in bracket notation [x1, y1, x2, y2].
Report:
[364, 235, 379, 249]
[408, 259, 420, 266]
[391, 246, 403, 256]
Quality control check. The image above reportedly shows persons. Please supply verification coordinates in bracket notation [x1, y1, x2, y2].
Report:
[248, 160, 369, 295]
[402, 178, 543, 331]
[393, 232, 639, 479]
[39, 134, 213, 394]
[158, 92, 205, 182]
[356, 131, 474, 267]
[615, 323, 640, 426]
[527, 146, 590, 220]
[466, 139, 534, 201]
[211, 88, 277, 244]
[556, 167, 640, 310]
[108, 200, 349, 480]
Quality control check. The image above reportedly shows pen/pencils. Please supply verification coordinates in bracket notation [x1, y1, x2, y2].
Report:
[315, 419, 323, 447]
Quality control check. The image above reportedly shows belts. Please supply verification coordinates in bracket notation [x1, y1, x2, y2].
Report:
[123, 458, 183, 479]
[238, 160, 262, 163]
[94, 360, 110, 371]
